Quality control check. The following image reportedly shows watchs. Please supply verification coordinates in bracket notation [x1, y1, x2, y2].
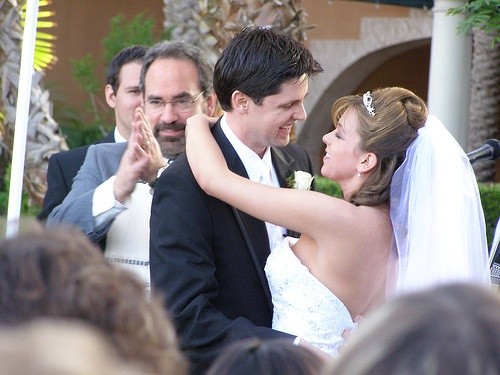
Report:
[148, 165, 171, 188]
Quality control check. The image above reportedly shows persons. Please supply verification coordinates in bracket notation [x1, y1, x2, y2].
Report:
[45, 40, 218, 301]
[0, 212, 500, 375]
[33, 45, 146, 257]
[150, 28, 332, 375]
[185, 86, 490, 357]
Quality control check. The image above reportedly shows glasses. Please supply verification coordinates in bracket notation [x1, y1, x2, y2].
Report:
[143, 91, 204, 113]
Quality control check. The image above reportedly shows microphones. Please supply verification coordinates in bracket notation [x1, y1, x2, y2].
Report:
[466, 139, 500, 163]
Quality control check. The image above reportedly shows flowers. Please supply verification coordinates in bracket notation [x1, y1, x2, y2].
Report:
[285, 168, 313, 191]
